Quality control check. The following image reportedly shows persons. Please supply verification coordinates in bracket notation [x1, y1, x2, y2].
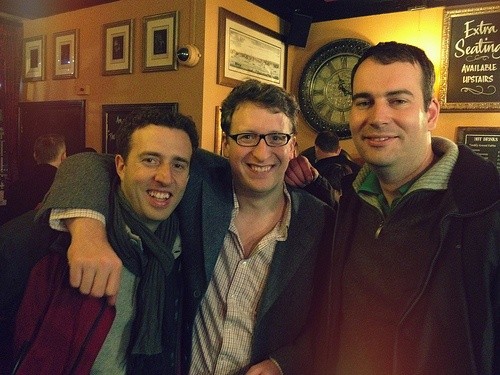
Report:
[6, 133, 67, 221]
[282, 42, 500, 375]
[34, 81, 336, 374]
[305, 131, 353, 203]
[1, 106, 199, 375]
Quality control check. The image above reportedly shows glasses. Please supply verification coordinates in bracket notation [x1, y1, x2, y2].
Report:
[226, 132, 295, 147]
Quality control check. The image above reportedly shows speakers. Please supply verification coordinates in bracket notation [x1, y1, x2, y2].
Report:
[285, 11, 312, 48]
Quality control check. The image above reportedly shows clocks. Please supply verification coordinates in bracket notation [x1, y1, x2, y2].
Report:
[295, 37, 371, 139]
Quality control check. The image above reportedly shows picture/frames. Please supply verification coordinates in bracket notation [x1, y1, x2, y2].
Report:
[142, 9, 178, 72]
[454, 126, 500, 172]
[101, 103, 180, 155]
[214, 105, 224, 156]
[215, 6, 288, 89]
[437, 4, 500, 114]
[102, 18, 133, 75]
[22, 34, 46, 82]
[53, 28, 79, 80]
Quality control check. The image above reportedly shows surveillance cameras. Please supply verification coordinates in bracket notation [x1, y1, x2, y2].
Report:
[177, 44, 200, 67]
